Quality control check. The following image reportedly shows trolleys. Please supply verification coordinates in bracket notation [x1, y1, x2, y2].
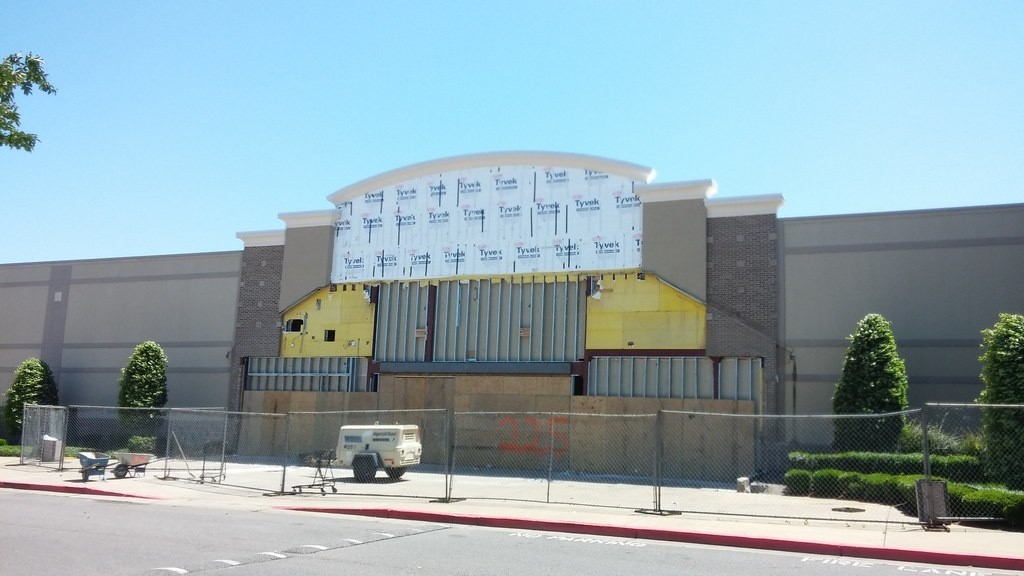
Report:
[77, 451, 122, 481]
[111, 452, 173, 479]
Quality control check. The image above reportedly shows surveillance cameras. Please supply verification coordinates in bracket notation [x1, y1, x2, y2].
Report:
[348, 342, 355, 346]
